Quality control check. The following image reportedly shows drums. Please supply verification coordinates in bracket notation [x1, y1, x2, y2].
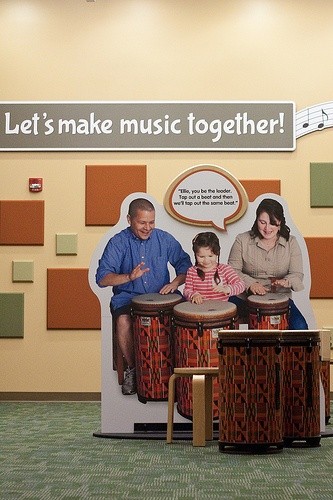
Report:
[128, 291, 183, 399]
[174, 298, 238, 424]
[245, 291, 291, 331]
[214, 328, 323, 454]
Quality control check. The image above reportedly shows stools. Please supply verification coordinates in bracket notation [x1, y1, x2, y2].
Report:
[166, 367, 219, 447]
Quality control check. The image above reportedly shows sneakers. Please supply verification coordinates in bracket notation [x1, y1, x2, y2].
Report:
[121, 365, 138, 395]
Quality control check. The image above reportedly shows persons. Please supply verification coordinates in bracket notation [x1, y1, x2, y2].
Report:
[228, 200, 309, 332]
[183, 231, 245, 306]
[96, 197, 193, 396]
